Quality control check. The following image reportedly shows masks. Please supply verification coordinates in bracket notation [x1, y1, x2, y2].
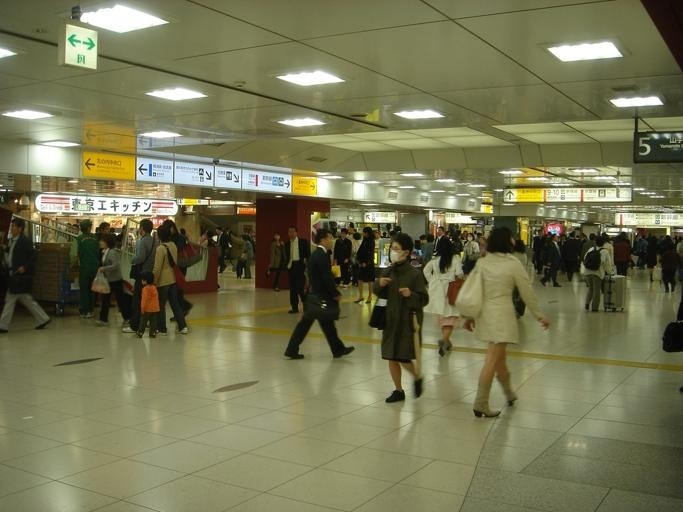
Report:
[388, 250, 406, 264]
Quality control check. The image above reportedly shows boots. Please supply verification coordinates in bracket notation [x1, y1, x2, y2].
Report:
[473, 375, 501, 418]
[497, 372, 518, 406]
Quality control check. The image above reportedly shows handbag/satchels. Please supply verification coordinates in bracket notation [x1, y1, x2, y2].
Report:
[129, 264, 142, 279]
[172, 264, 189, 290]
[368, 288, 388, 331]
[305, 293, 339, 320]
[662, 321, 682, 352]
[447, 278, 465, 305]
[178, 242, 203, 268]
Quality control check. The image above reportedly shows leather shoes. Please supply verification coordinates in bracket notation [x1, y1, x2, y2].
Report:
[334, 346, 355, 358]
[386, 390, 405, 403]
[293, 354, 304, 359]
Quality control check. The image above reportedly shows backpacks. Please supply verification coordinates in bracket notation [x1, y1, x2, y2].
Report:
[583, 246, 606, 271]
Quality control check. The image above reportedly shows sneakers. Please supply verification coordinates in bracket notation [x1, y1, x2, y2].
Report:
[437, 338, 452, 358]
[0, 328, 9, 333]
[80, 303, 192, 338]
[36, 319, 52, 330]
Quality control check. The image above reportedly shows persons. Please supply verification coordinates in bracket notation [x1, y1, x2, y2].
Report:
[532, 229, 682, 311]
[1, 218, 52, 333]
[374, 224, 552, 418]
[267, 221, 375, 358]
[67, 218, 193, 337]
[200, 228, 254, 278]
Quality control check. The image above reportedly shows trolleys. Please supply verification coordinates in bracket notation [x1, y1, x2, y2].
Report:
[56, 257, 84, 316]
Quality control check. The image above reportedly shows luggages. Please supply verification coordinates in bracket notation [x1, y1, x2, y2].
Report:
[602, 273, 626, 312]
